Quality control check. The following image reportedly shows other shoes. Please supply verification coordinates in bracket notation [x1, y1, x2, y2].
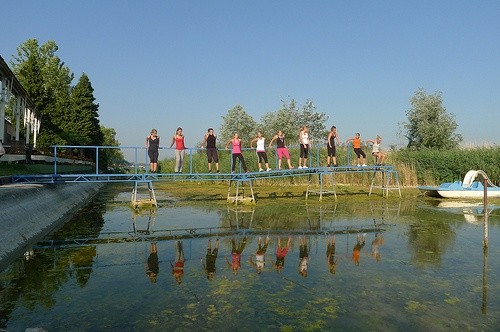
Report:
[150, 171, 157, 175]
[278, 168, 283, 170]
[216, 170, 221, 174]
[232, 171, 236, 174]
[245, 171, 250, 174]
[267, 168, 272, 172]
[376, 164, 383, 167]
[357, 164, 367, 167]
[175, 172, 182, 174]
[327, 165, 337, 167]
[259, 168, 264, 172]
[209, 171, 212, 174]
[298, 166, 309, 169]
[289, 166, 295, 170]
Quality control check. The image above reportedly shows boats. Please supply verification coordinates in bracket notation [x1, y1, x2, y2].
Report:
[418, 169, 500, 199]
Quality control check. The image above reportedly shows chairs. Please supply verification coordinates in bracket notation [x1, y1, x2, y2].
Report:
[454, 181, 462, 187]
[471, 182, 482, 189]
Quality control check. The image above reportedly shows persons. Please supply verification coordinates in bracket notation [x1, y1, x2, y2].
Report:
[144, 243, 161, 283]
[201, 129, 220, 173]
[200, 239, 219, 280]
[298, 127, 310, 169]
[252, 132, 272, 172]
[269, 131, 294, 170]
[366, 137, 383, 165]
[248, 235, 270, 275]
[226, 134, 249, 173]
[170, 128, 188, 174]
[268, 236, 292, 272]
[146, 129, 159, 173]
[297, 235, 310, 277]
[327, 126, 340, 167]
[344, 234, 367, 266]
[168, 240, 185, 284]
[346, 133, 367, 166]
[326, 234, 338, 275]
[224, 238, 248, 276]
[368, 232, 384, 261]
